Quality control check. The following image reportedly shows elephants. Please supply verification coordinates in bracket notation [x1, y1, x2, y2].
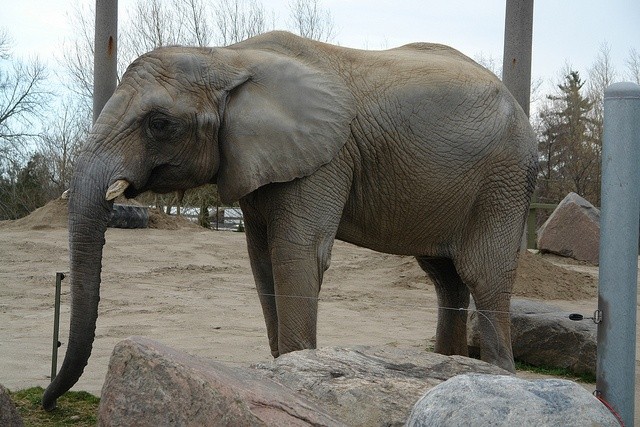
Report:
[42, 30, 537, 416]
